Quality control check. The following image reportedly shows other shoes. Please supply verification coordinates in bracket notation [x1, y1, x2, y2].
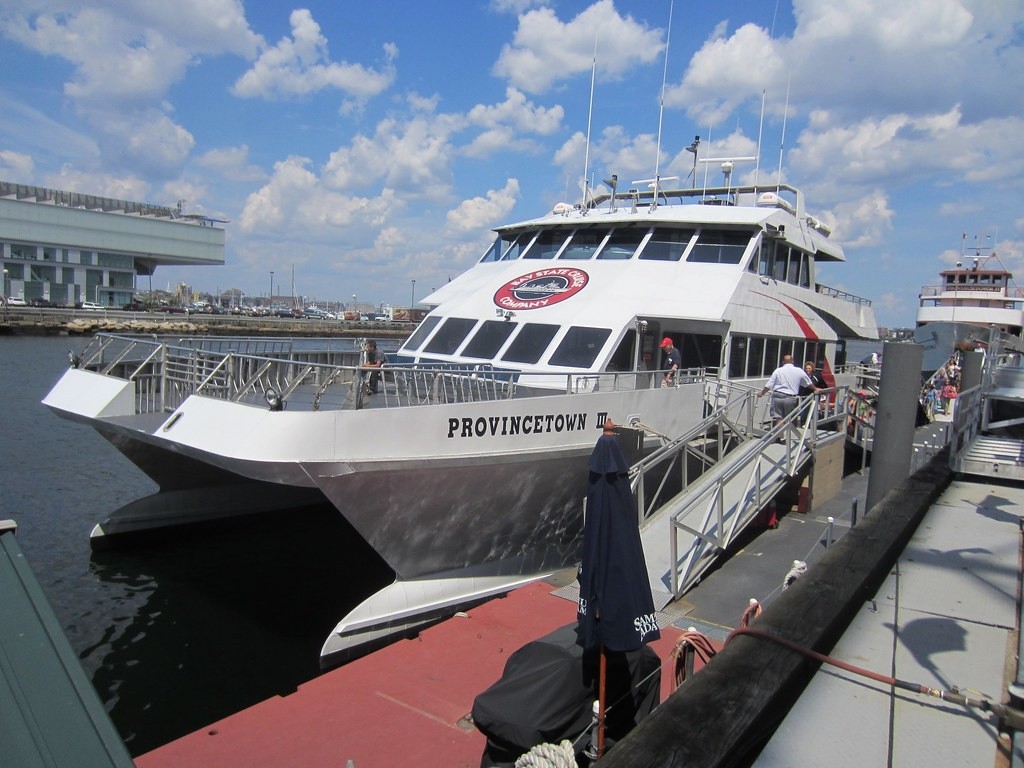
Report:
[366, 388, 374, 395]
[776, 436, 786, 444]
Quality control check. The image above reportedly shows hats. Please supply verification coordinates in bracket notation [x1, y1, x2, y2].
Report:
[659, 338, 673, 348]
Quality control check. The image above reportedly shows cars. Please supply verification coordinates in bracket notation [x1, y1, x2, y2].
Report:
[0, 296, 388, 327]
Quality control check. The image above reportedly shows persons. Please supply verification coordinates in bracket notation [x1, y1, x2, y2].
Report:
[660, 337, 682, 386]
[756, 354, 824, 444]
[973, 342, 986, 371]
[922, 355, 964, 423]
[843, 385, 877, 442]
[796, 360, 830, 430]
[353, 339, 391, 394]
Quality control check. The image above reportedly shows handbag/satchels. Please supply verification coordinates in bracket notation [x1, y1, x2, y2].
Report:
[943, 384, 958, 398]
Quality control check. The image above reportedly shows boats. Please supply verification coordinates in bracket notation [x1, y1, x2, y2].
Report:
[840, 221, 1024, 470]
[34, 2, 890, 671]
